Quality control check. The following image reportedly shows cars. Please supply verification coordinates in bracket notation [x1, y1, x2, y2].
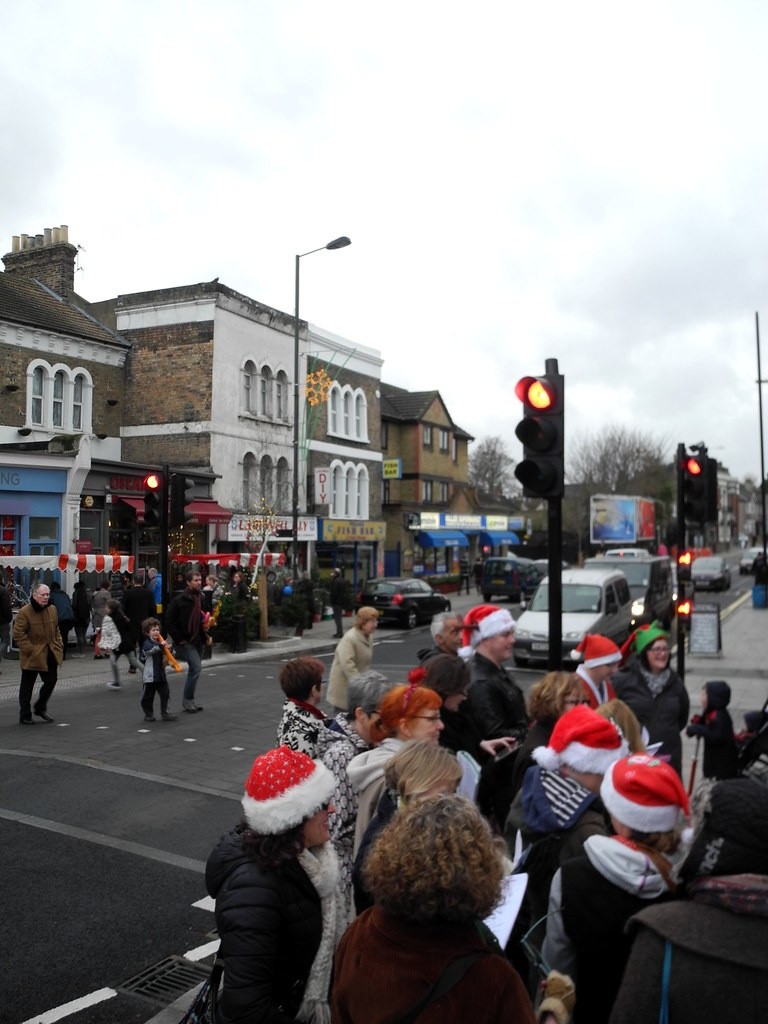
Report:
[669, 561, 695, 608]
[354, 575, 451, 630]
[692, 555, 732, 590]
[739, 546, 768, 575]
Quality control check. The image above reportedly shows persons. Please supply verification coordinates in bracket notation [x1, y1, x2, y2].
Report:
[139, 618, 179, 721]
[12, 583, 63, 725]
[230, 571, 247, 599]
[205, 604, 768, 1024]
[97, 599, 145, 690]
[282, 572, 316, 629]
[471, 557, 484, 594]
[0, 568, 13, 674]
[205, 575, 224, 607]
[656, 540, 668, 555]
[50, 566, 187, 674]
[164, 570, 207, 713]
[329, 567, 345, 638]
[456, 555, 471, 596]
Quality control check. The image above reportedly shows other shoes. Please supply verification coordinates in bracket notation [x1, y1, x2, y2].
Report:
[144, 714, 156, 721]
[94, 654, 104, 660]
[128, 668, 136, 674]
[192, 699, 204, 711]
[33, 709, 54, 722]
[71, 653, 86, 658]
[106, 682, 123, 690]
[333, 633, 345, 639]
[162, 710, 179, 721]
[19, 717, 35, 724]
[104, 654, 110, 659]
[182, 699, 197, 714]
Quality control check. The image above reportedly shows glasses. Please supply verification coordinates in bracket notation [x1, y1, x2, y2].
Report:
[562, 698, 594, 708]
[458, 690, 469, 700]
[519, 905, 566, 981]
[321, 799, 333, 811]
[416, 715, 444, 722]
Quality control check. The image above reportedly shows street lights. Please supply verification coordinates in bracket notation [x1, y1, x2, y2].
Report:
[293, 236, 352, 579]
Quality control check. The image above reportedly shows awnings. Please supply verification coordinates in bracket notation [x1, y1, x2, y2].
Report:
[0, 555, 135, 574]
[419, 531, 470, 549]
[479, 531, 519, 545]
[120, 498, 232, 523]
[172, 553, 286, 567]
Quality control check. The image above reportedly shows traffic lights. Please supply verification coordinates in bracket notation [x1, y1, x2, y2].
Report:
[679, 456, 711, 524]
[677, 550, 692, 581]
[169, 473, 195, 523]
[143, 472, 164, 527]
[678, 599, 691, 633]
[514, 375, 564, 499]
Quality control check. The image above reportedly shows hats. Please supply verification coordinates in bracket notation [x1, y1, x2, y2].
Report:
[456, 604, 519, 664]
[599, 751, 698, 844]
[617, 617, 673, 670]
[238, 743, 345, 835]
[530, 703, 634, 775]
[568, 633, 625, 670]
[666, 775, 768, 888]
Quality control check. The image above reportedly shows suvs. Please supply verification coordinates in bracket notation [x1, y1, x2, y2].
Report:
[479, 556, 545, 603]
[513, 566, 633, 668]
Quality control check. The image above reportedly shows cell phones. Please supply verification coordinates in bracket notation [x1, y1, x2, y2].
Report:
[493, 738, 524, 762]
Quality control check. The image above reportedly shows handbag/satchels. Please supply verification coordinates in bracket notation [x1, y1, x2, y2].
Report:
[178, 975, 220, 1024]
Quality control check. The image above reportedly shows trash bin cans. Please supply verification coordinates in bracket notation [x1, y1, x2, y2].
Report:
[753, 583, 768, 607]
[173, 590, 215, 660]
[232, 615, 249, 653]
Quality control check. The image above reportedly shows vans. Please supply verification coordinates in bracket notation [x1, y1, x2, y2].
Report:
[582, 548, 676, 632]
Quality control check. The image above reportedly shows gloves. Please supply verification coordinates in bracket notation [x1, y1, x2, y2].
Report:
[691, 714, 702, 723]
[685, 724, 707, 739]
